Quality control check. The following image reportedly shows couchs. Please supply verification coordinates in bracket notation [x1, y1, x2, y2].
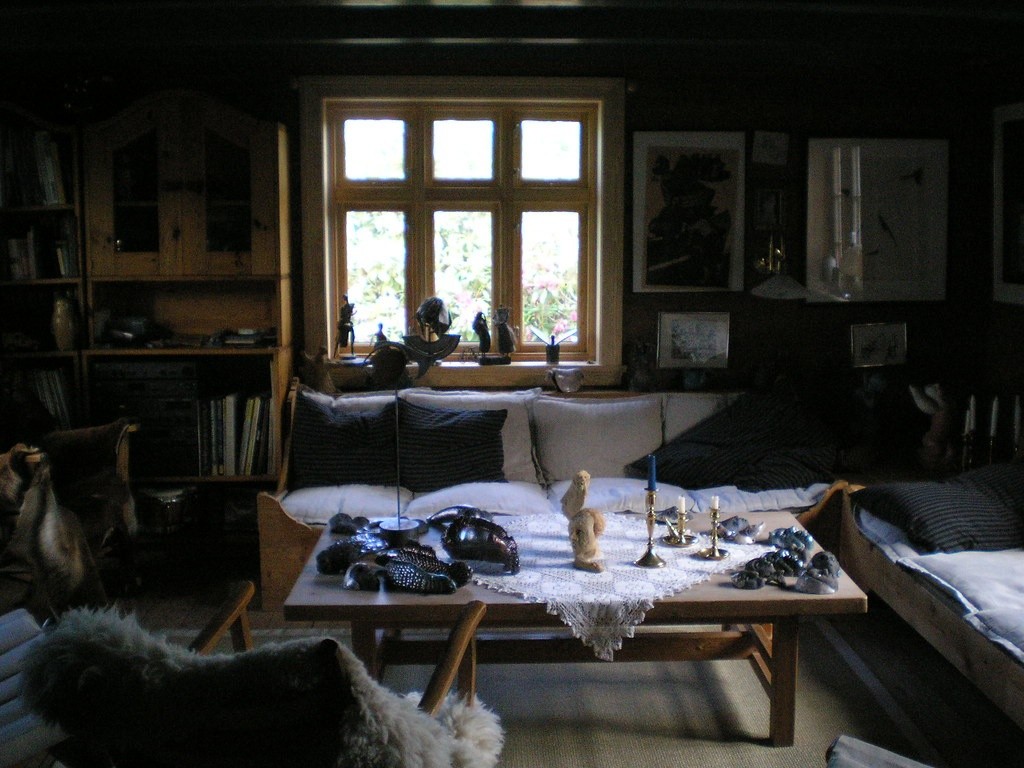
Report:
[275, 389, 864, 561]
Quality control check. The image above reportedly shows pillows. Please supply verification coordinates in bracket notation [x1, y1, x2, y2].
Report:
[396, 397, 512, 494]
[286, 394, 395, 490]
[737, 394, 857, 493]
[630, 405, 787, 490]
[407, 386, 541, 489]
[852, 461, 1024, 552]
[533, 398, 662, 487]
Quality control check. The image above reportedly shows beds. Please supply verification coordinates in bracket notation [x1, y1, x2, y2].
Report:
[841, 468, 1024, 729]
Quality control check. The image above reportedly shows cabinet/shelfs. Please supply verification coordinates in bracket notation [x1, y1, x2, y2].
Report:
[83, 93, 307, 491]
[0, 104, 82, 449]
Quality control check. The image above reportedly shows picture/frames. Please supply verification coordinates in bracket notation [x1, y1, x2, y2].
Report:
[808, 139, 951, 304]
[628, 125, 757, 292]
[993, 105, 1024, 304]
[656, 307, 732, 368]
[850, 321, 909, 370]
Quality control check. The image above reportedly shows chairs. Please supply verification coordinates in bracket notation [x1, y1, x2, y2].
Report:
[19, 579, 502, 768]
[0, 382, 139, 616]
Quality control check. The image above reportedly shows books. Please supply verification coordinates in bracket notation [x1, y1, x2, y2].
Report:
[0, 132, 77, 281]
[203, 395, 269, 476]
[34, 366, 75, 431]
[224, 335, 259, 344]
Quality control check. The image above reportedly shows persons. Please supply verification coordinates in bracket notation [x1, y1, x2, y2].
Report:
[333, 294, 355, 358]
[567, 508, 606, 561]
[369, 323, 386, 350]
[473, 312, 491, 357]
[492, 303, 518, 358]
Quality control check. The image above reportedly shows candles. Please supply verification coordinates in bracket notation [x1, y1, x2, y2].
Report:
[970, 395, 977, 428]
[1013, 395, 1021, 448]
[990, 398, 997, 438]
[963, 410, 972, 434]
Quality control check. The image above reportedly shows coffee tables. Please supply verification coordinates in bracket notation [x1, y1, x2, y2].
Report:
[290, 503, 868, 746]
